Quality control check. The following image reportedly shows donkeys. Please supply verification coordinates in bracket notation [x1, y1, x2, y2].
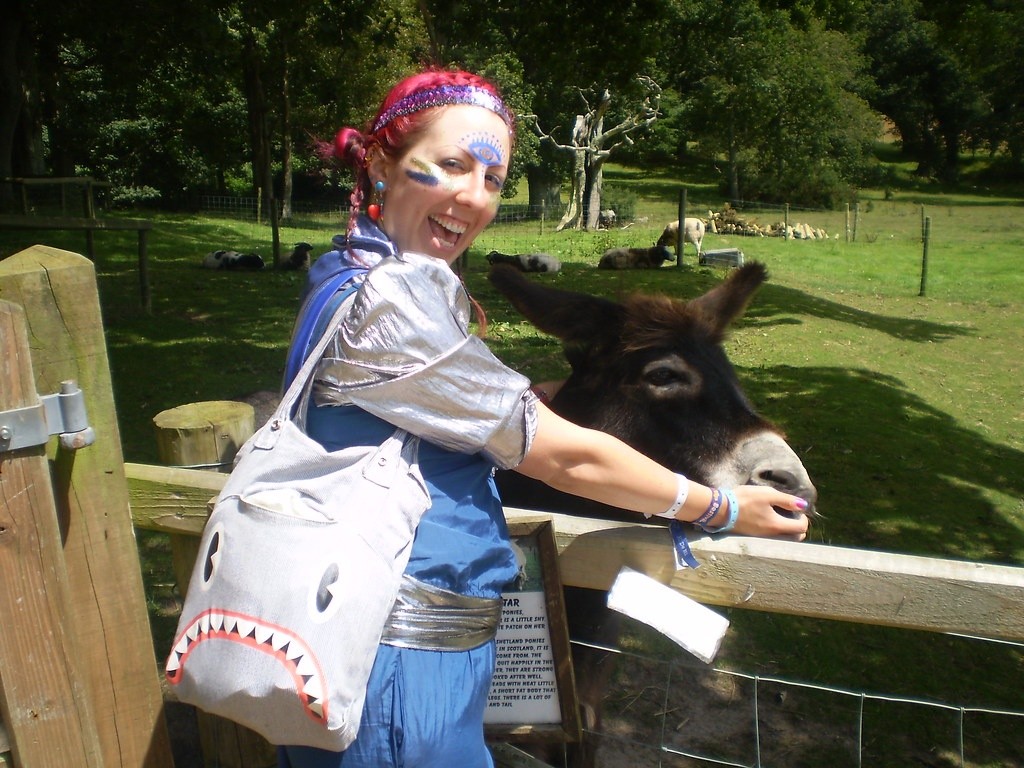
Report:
[487, 261, 818, 768]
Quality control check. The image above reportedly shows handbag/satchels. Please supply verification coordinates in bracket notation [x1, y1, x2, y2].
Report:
[164, 291, 431, 754]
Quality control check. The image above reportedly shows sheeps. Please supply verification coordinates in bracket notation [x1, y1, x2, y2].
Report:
[653, 218, 705, 257]
[486, 245, 675, 272]
[203, 241, 314, 272]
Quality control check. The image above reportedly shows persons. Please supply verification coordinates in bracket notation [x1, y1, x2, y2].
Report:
[273, 65, 809, 768]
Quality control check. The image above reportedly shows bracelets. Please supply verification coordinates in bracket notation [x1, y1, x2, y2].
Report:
[667, 486, 723, 572]
[700, 486, 738, 533]
[643, 472, 690, 521]
